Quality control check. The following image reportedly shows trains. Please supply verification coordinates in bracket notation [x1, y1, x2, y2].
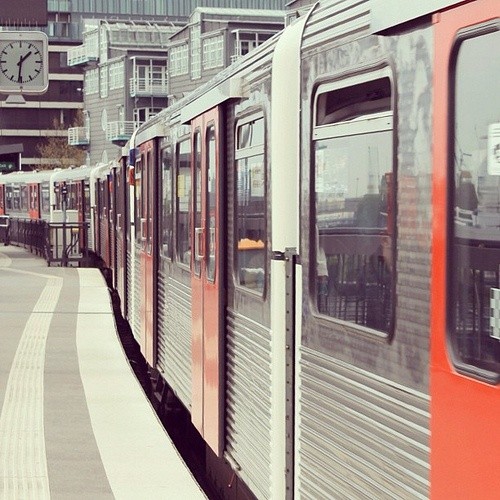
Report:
[0, 0, 499, 500]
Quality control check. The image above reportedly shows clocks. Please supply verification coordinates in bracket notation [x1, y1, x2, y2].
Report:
[0, 31, 51, 95]
